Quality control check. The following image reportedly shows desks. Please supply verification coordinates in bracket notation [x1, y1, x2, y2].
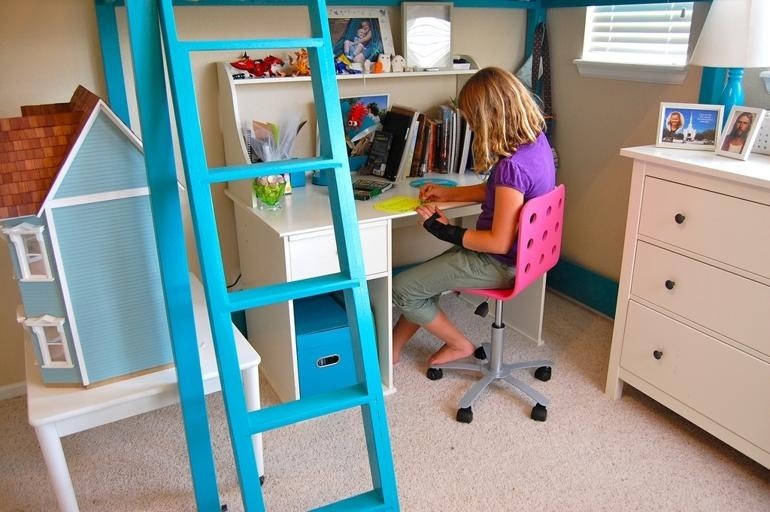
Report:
[24, 271, 265, 512]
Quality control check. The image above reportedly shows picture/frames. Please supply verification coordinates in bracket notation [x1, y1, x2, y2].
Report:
[713, 104, 767, 162]
[326, 6, 396, 72]
[655, 101, 725, 152]
[401, 1, 454, 71]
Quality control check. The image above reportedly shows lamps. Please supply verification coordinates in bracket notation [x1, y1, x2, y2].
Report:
[687, 1, 770, 131]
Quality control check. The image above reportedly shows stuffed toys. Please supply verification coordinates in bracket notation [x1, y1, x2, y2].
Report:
[346, 103, 370, 131]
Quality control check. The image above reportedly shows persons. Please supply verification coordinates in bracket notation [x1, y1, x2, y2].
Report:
[392, 66, 555, 366]
[667, 113, 682, 133]
[344, 29, 367, 62]
[343, 20, 372, 63]
[721, 112, 753, 154]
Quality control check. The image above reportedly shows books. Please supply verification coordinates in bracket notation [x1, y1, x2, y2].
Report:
[364, 104, 475, 182]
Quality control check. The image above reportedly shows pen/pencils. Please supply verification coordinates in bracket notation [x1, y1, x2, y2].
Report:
[421, 186, 433, 205]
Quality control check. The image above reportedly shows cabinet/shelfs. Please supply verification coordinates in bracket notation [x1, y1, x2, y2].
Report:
[217, 54, 548, 404]
[604, 144, 769, 472]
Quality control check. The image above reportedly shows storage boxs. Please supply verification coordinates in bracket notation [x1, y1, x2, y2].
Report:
[292, 293, 364, 400]
